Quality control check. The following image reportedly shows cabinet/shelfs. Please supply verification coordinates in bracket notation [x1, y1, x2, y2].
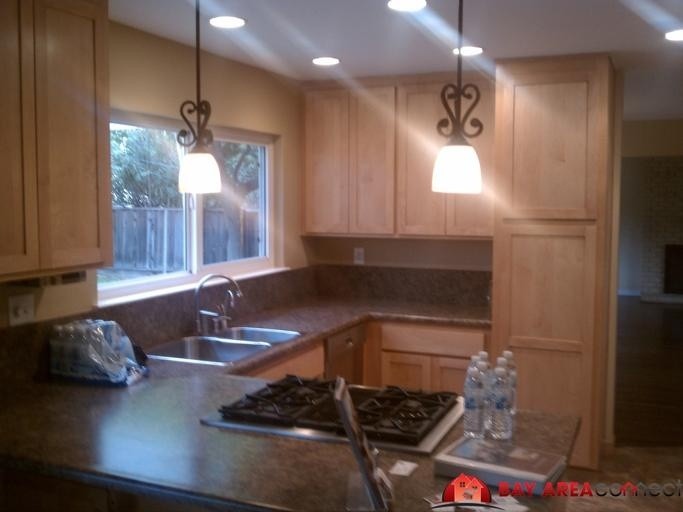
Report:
[1, 0, 115, 290]
[492, 53, 618, 226]
[363, 318, 485, 402]
[397, 70, 492, 242]
[298, 75, 397, 240]
[491, 226, 610, 472]
[322, 319, 370, 388]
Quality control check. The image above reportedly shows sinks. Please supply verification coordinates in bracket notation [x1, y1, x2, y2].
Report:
[211, 325, 306, 342]
[146, 336, 270, 367]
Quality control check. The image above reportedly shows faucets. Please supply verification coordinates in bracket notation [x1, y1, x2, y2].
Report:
[223, 289, 235, 315]
[194, 274, 243, 335]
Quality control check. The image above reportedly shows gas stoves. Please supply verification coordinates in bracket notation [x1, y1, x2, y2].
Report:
[198, 372, 467, 454]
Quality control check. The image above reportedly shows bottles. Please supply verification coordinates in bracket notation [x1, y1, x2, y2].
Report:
[463, 348, 518, 439]
[50, 318, 116, 379]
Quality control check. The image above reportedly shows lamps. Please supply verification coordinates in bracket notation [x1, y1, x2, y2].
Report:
[425, 0, 485, 197]
[171, 1, 228, 199]
